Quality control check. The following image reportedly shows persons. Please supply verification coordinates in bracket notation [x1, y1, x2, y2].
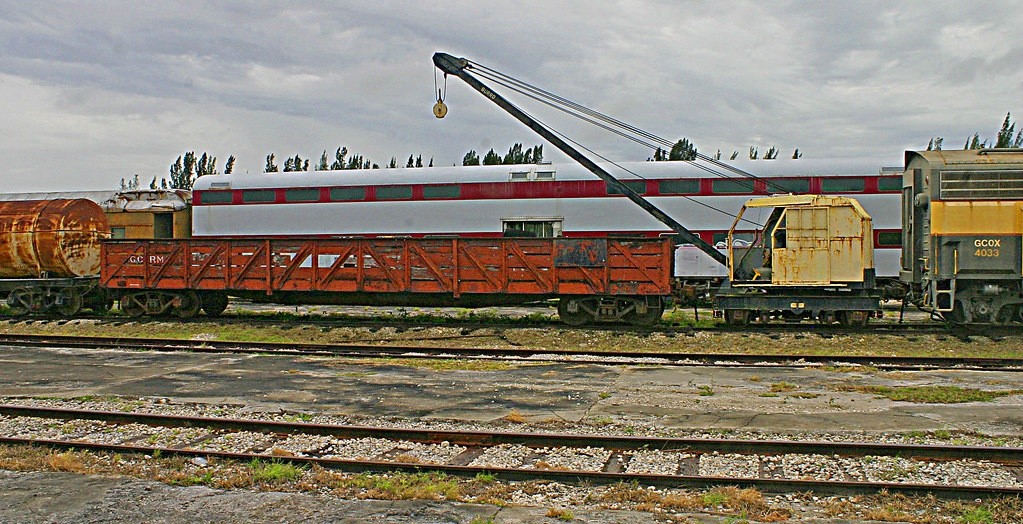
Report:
[769, 221, 786, 246]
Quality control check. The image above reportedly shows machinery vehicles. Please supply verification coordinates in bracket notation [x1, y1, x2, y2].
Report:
[427, 50, 879, 294]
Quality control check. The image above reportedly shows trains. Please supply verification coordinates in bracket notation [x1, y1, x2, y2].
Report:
[191, 159, 905, 281]
[0, 135, 1023, 332]
